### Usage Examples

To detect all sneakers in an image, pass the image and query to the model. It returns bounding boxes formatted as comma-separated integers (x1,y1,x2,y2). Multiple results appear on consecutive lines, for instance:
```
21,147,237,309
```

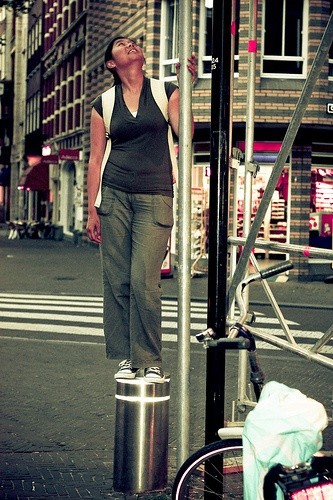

144,367,164,383
115,360,139,378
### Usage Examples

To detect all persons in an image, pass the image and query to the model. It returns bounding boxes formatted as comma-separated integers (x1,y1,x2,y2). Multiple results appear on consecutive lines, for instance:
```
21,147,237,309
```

85,37,199,384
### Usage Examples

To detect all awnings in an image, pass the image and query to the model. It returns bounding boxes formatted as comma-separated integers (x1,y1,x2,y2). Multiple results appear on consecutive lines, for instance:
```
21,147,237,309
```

17,161,50,191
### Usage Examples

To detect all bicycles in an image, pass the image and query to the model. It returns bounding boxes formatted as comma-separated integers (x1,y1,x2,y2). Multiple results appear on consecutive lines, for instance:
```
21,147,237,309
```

171,259,333,500
